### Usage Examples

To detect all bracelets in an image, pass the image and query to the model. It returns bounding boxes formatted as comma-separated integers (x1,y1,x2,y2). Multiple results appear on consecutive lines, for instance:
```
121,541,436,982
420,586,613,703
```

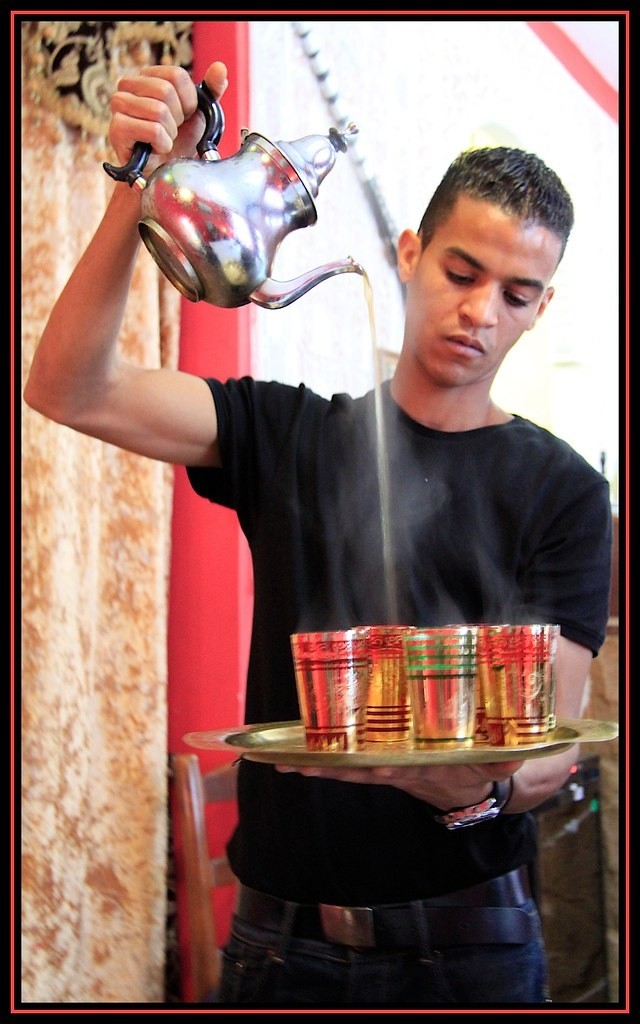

500,776,514,811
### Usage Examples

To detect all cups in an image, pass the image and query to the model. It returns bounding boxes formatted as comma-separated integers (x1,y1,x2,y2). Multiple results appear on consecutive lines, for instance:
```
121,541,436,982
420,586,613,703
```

289,621,561,754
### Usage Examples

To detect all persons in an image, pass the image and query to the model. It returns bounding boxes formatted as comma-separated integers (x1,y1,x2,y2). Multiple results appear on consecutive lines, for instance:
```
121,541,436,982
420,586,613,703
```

24,61,614,1003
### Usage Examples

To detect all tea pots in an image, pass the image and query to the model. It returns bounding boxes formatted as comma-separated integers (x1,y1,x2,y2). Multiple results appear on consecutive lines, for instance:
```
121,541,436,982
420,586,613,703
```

103,81,365,310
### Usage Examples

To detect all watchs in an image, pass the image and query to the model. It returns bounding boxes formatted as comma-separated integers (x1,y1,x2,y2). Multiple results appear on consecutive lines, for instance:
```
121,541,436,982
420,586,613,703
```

436,780,506,831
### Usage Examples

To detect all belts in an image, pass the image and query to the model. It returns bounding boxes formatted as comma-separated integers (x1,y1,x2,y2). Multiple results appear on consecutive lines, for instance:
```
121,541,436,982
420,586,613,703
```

233,863,533,951
